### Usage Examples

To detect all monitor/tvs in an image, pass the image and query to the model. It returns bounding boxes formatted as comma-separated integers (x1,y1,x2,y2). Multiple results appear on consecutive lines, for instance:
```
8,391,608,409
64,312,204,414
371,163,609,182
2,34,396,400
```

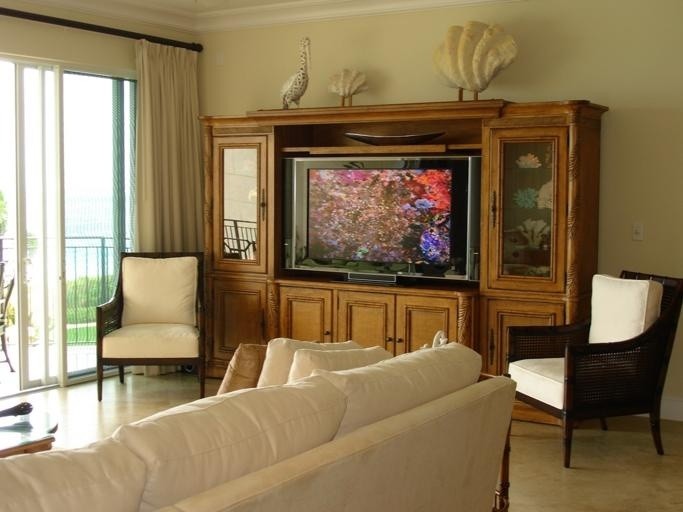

280,156,480,288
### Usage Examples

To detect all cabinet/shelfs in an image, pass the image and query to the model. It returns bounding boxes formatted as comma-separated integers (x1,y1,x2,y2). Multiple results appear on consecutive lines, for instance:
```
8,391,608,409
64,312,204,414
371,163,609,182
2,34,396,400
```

199,98,609,429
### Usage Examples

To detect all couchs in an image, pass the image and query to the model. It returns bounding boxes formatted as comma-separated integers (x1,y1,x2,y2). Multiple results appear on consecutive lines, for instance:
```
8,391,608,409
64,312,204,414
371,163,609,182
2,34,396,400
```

1,336,516,508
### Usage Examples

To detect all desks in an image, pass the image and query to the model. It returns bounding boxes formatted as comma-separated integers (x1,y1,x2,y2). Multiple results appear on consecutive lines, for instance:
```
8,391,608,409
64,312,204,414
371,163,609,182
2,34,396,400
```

2,408,53,464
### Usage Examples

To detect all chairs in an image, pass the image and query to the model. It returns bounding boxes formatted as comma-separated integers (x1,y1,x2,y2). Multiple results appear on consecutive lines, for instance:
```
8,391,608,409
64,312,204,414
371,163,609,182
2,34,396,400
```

94,249,209,408
497,269,683,468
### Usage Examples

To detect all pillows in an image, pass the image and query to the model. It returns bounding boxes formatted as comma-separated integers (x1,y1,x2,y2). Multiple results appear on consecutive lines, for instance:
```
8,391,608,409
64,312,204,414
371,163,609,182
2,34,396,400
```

211,338,392,407
